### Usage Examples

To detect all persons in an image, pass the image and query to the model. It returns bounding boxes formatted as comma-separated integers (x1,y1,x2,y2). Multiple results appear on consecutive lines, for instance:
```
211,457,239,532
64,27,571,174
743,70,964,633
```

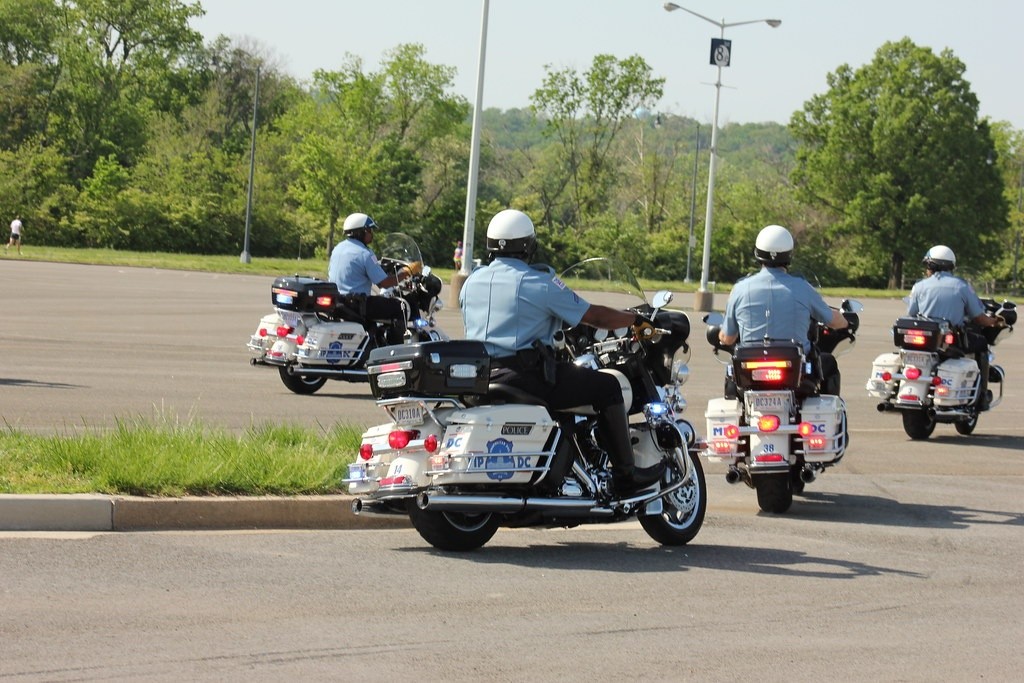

327,213,421,346
907,245,999,412
6,215,23,255
718,224,849,400
459,209,667,499
453,241,462,273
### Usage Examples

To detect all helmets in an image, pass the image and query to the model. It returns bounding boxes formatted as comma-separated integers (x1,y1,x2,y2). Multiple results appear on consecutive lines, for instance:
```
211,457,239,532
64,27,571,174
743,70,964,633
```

343,213,378,231
486,209,537,251
922,245,956,271
754,225,794,263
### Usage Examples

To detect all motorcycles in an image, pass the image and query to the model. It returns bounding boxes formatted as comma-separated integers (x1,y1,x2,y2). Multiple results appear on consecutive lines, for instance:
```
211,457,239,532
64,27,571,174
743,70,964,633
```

248,232,451,396
340,255,708,554
866,266,1019,439
702,264,865,515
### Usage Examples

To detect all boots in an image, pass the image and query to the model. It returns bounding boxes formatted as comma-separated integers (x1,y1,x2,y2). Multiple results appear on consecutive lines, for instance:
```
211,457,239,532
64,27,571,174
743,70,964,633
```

976,352,993,411
598,403,666,496
821,371,849,448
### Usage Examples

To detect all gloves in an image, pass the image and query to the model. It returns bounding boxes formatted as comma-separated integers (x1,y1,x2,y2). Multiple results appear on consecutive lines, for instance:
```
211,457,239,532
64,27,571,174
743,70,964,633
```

403,261,422,277
991,316,1005,329
633,312,656,340
843,312,860,333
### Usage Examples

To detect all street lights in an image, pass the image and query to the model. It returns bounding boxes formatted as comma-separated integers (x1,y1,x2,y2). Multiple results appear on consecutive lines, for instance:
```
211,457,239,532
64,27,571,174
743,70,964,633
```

664,1,782,311
